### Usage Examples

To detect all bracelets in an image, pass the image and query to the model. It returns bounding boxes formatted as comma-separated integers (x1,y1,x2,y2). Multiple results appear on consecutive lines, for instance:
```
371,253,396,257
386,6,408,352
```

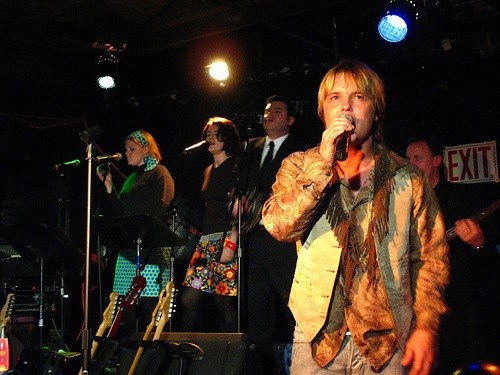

224,240,237,251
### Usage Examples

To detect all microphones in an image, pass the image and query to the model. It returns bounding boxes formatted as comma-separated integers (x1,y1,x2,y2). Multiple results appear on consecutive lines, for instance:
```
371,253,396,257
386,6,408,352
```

95,153,123,164
53,159,81,170
244,127,252,149
183,139,210,154
336,114,354,160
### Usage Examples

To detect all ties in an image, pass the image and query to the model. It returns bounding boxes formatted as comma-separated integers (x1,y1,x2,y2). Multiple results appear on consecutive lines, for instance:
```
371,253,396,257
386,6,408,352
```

259,140,274,175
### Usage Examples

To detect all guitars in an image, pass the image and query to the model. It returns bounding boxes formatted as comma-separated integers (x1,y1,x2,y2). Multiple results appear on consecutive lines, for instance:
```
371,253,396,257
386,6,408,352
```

0,275,178,375
444,199,500,241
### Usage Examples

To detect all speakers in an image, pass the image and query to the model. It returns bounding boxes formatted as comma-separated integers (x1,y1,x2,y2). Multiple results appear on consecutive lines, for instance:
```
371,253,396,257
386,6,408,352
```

13,348,82,375
115,331,266,375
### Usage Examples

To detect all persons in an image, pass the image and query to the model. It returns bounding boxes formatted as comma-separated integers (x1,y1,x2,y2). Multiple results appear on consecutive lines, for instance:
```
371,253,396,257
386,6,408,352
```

405,138,500,375
262,62,451,375
180,116,247,331
95,130,175,332
241,93,307,374
